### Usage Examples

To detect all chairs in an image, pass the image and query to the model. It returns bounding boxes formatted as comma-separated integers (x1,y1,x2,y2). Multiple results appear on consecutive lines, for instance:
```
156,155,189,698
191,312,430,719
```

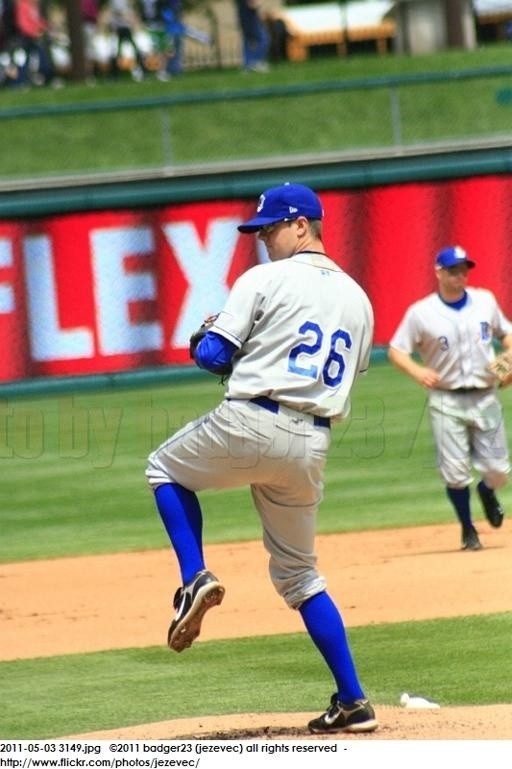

346,16,397,57
268,5,347,61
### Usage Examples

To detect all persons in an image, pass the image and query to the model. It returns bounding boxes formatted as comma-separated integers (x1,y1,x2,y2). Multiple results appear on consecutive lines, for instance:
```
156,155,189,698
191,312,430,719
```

387,244,512,551
145,182,381,734
0,0,186,88
238,0,293,78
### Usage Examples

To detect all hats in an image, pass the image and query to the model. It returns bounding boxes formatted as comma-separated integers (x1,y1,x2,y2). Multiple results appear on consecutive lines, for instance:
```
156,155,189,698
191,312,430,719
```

435,244,475,271
237,183,324,234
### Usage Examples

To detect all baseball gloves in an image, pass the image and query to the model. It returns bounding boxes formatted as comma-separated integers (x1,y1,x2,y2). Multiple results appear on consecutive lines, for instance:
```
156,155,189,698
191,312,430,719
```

486,352,512,388
189,313,230,385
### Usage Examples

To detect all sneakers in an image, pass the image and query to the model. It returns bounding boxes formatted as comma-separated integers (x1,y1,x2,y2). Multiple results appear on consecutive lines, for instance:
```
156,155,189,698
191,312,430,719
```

476,480,504,529
167,569,225,653
308,693,378,735
461,525,482,550
